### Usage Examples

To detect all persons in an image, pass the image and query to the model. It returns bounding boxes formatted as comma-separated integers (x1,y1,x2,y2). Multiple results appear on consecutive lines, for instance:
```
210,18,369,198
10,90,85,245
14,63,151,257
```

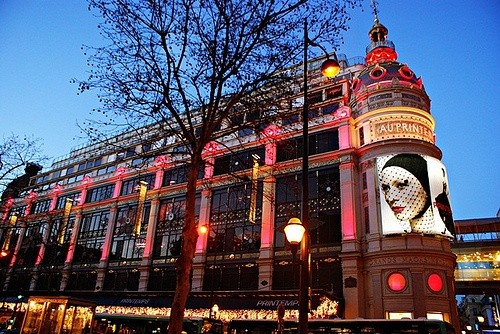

2,300,224,334
436,167,454,238
379,154,434,235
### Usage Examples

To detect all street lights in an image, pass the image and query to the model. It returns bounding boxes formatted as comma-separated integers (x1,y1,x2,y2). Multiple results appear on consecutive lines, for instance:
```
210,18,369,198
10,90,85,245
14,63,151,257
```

283,16,340,334
